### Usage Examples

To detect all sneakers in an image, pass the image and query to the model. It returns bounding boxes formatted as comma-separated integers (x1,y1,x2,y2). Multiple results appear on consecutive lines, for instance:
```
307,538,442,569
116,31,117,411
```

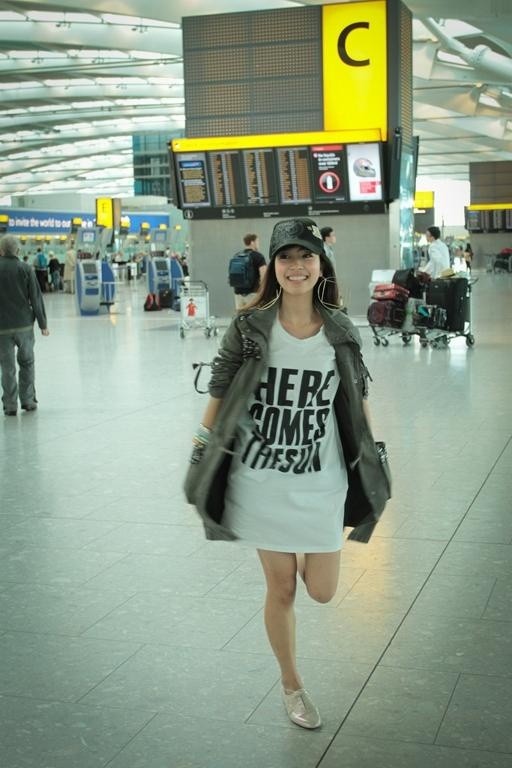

22,402,37,410
5,411,17,416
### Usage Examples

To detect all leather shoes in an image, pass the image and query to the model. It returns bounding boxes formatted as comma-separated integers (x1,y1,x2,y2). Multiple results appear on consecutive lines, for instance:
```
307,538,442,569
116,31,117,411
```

281,683,322,731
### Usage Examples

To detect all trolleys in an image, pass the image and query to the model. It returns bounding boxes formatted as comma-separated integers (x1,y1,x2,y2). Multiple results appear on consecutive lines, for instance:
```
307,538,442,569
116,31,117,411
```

174,278,220,338
369,323,414,346
414,273,480,349
484,251,505,274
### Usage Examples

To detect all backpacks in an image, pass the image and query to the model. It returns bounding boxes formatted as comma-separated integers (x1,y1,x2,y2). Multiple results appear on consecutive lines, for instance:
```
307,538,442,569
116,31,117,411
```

228,248,256,295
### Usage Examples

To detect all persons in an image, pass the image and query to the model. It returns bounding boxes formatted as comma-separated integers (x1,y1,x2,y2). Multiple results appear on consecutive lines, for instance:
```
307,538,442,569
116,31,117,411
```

0,232,49,417
319,225,337,277
184,218,396,730
21,241,191,295
421,243,473,274
233,233,268,318
418,225,453,281
185,298,199,316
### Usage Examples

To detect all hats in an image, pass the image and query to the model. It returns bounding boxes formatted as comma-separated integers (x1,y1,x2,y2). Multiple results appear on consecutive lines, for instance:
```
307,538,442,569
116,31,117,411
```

440,268,459,278
268,216,326,260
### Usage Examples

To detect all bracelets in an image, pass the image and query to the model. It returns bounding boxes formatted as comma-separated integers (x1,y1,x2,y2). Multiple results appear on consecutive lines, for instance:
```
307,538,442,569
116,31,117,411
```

198,422,214,434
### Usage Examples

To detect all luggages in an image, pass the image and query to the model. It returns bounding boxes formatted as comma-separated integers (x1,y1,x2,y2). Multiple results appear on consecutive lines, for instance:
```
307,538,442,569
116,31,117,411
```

366,266,469,334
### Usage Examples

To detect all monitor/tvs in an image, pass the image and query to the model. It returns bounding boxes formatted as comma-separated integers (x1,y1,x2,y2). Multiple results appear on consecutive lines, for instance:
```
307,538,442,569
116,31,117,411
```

155,229,166,241
155,261,167,271
82,264,97,273
81,229,96,243
